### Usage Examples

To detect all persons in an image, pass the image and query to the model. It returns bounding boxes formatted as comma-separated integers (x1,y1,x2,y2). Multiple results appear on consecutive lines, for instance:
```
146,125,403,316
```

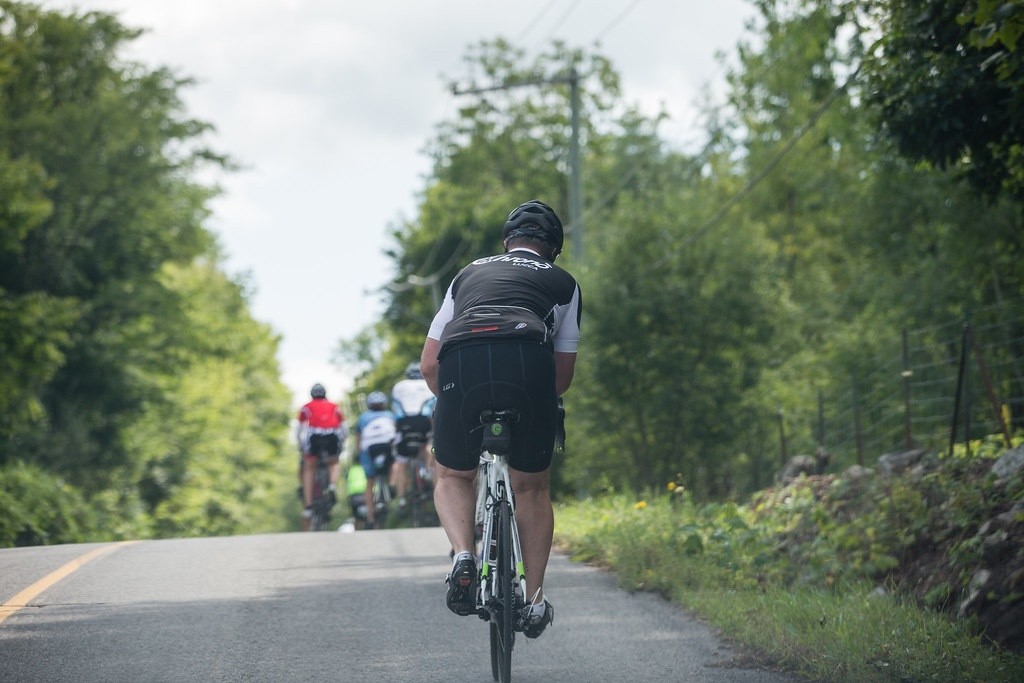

346,361,436,526
419,199,582,639
295,383,350,518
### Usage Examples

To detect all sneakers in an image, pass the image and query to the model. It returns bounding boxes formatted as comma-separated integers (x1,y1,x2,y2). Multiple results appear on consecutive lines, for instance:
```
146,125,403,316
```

444,551,476,618
522,597,554,639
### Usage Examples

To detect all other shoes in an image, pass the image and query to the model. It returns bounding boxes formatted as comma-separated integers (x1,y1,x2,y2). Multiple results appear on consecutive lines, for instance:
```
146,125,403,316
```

302,510,315,521
362,514,374,529
419,468,436,482
398,497,408,507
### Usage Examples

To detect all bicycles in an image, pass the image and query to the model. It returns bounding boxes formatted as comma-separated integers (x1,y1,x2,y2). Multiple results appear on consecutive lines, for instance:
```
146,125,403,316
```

367,430,431,530
453,408,528,683
303,451,337,532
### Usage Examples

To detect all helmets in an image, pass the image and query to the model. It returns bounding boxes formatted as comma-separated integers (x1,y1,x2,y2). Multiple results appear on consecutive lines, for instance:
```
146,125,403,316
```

366,391,390,411
312,383,327,400
406,361,425,381
501,199,563,252
